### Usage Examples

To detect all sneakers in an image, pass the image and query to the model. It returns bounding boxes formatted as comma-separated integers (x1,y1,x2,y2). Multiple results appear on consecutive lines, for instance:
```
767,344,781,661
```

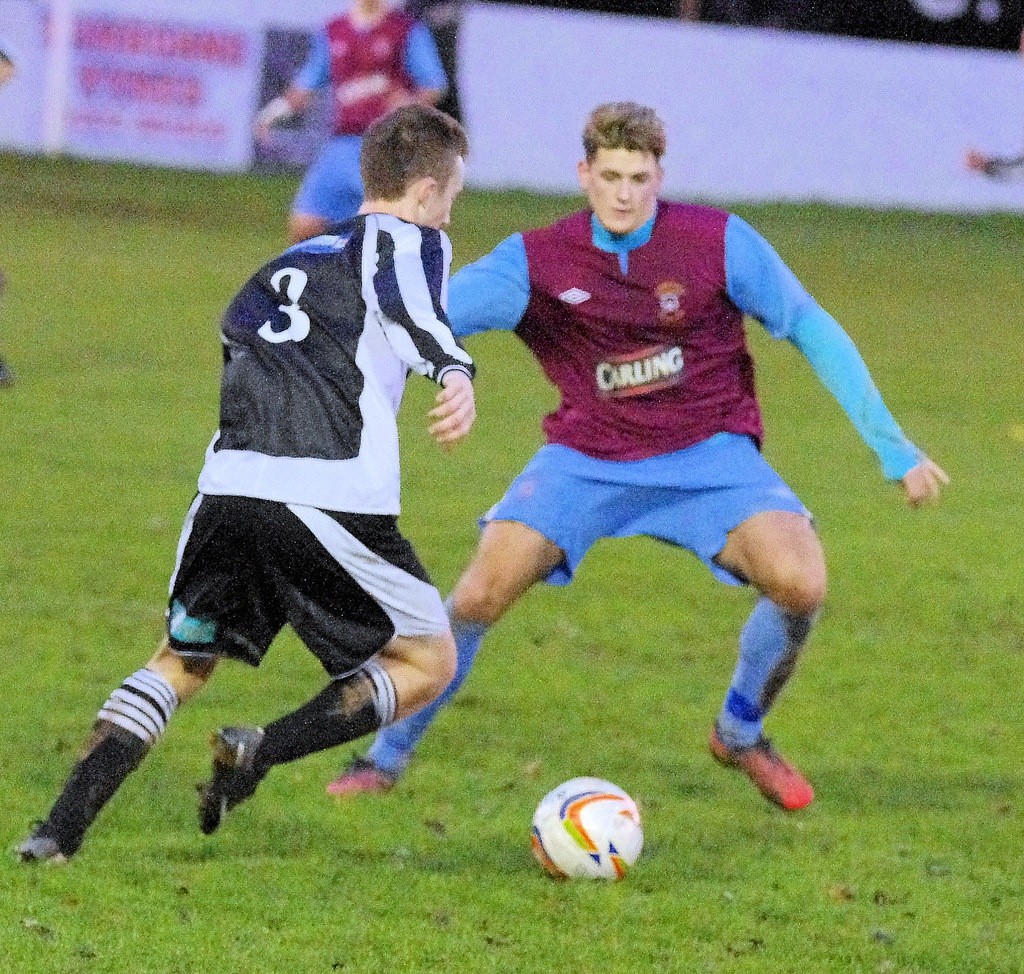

192,724,266,834
708,720,815,812
325,751,396,799
11,819,68,865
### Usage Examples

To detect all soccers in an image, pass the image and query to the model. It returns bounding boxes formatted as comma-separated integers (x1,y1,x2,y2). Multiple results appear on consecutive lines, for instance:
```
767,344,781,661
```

525,773,646,882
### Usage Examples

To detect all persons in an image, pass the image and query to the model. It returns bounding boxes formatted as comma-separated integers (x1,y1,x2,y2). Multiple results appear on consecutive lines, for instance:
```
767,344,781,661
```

0,49,14,386
323,103,952,810
964,149,1024,182
253,0,447,247
17,104,477,866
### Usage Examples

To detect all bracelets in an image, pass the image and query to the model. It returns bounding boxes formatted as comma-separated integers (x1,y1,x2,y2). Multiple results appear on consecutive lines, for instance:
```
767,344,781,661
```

259,97,294,125
